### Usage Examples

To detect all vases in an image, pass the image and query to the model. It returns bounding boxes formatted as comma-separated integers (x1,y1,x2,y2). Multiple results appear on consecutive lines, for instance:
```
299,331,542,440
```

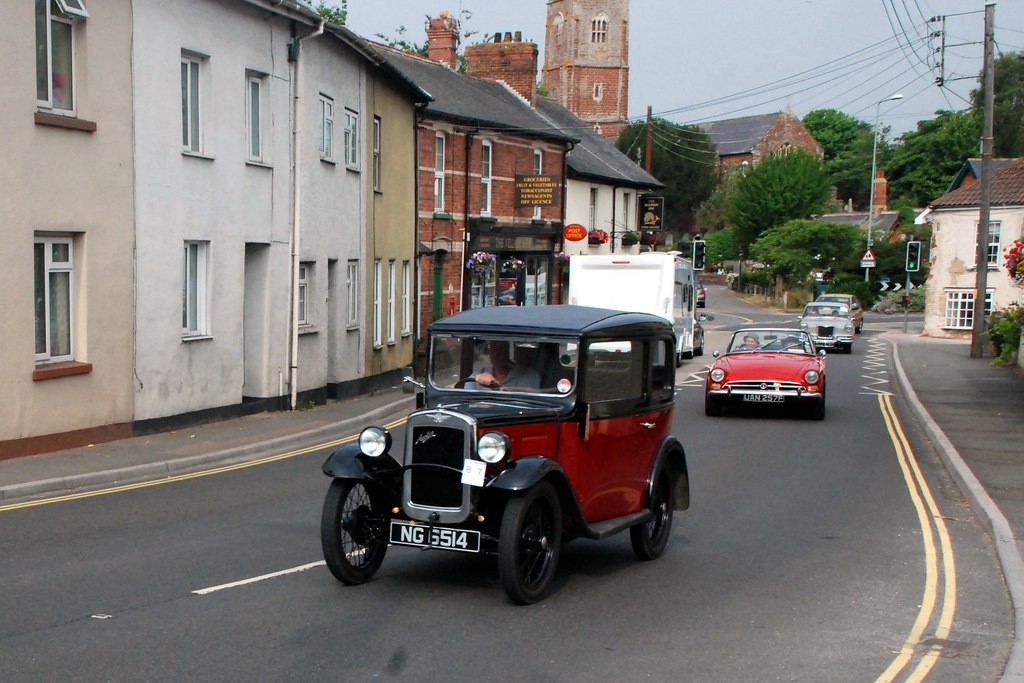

588,238,605,245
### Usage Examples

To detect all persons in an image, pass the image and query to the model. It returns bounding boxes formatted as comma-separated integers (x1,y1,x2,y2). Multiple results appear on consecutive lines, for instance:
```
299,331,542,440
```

778,334,806,354
732,333,761,351
833,310,839,317
446,341,542,393
811,307,820,314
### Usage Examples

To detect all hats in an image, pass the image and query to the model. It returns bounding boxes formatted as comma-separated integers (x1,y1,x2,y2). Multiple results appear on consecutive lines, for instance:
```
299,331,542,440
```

744,332,759,344
781,336,804,346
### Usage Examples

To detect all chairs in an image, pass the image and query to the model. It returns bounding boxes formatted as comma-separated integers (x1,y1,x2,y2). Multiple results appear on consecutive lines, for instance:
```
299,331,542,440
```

505,346,640,402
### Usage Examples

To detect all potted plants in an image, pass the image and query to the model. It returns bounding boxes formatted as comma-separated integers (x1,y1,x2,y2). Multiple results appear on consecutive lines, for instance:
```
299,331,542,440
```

984,302,1024,368
621,230,641,245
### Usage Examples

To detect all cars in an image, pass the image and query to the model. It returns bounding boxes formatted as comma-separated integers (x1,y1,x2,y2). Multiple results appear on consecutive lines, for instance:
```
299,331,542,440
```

798,302,856,354
814,294,865,334
703,326,826,421
697,282,707,308
321,304,690,606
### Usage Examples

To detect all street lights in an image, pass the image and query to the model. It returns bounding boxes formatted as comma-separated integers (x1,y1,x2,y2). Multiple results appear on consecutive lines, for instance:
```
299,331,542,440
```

864,93,904,281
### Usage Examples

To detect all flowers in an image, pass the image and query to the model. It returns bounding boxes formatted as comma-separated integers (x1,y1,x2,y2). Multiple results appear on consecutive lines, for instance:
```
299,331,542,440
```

588,229,609,245
500,256,525,275
464,252,497,275
1001,238,1024,281
554,251,570,264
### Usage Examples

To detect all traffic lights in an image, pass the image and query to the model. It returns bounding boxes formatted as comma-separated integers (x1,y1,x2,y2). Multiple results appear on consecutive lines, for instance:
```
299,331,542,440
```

693,240,705,271
905,241,921,273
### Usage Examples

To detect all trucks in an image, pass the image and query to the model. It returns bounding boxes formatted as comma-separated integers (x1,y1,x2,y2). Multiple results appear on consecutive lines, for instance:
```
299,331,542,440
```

568,251,704,369
